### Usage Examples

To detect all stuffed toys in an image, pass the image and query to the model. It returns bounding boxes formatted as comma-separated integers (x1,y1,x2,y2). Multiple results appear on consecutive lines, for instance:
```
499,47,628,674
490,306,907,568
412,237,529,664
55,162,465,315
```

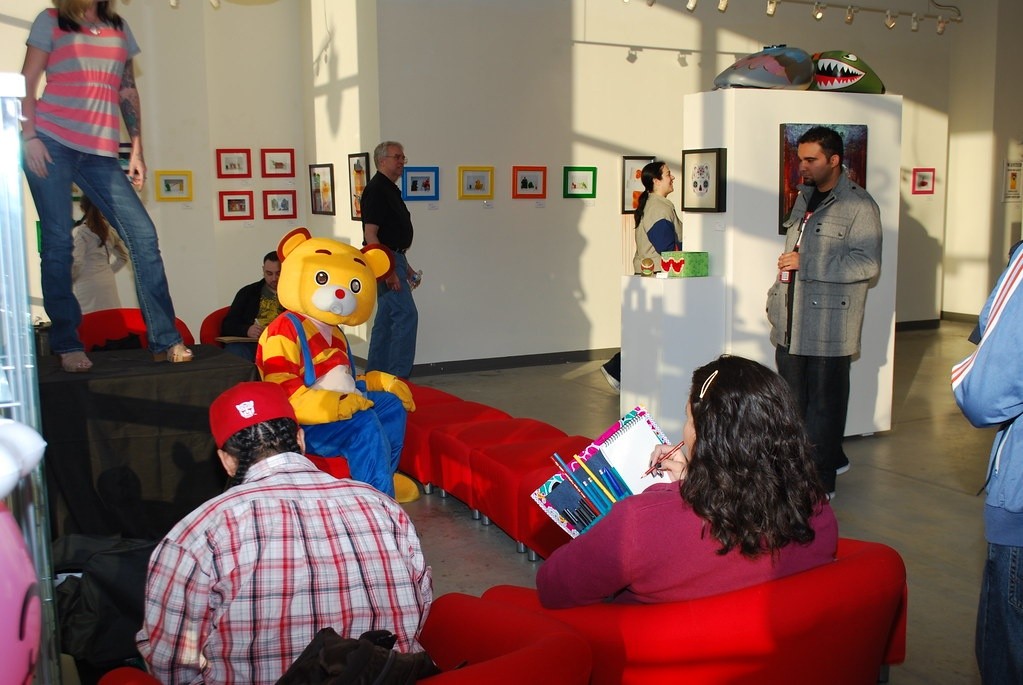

254,224,420,501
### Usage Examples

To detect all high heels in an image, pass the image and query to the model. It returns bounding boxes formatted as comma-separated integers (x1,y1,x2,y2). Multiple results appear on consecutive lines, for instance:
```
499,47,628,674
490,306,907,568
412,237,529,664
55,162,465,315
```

152,341,192,363
62,352,90,372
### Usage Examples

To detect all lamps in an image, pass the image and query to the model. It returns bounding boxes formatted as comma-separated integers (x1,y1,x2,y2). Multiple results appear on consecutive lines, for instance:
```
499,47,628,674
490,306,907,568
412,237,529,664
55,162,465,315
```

624,0,963,36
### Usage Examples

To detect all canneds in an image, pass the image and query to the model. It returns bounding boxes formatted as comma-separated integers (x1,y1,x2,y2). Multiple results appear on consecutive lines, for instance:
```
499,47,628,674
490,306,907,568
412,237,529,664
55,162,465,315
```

779,269,792,284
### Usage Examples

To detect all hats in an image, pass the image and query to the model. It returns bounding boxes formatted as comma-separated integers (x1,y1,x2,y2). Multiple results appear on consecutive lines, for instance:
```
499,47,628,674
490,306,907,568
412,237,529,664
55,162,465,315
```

209,381,300,450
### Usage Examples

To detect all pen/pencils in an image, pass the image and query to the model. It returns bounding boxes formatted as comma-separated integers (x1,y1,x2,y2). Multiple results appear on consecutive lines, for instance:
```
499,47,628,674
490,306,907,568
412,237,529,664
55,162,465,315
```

550,453,624,532
641,441,684,479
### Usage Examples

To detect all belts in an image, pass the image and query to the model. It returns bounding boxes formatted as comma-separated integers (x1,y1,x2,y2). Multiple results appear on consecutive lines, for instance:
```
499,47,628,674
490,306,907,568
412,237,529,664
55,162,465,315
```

394,249,406,254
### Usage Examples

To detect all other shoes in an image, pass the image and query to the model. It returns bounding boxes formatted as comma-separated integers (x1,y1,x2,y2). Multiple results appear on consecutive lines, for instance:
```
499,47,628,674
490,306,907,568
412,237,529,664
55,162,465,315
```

835,455,850,475
601,366,620,393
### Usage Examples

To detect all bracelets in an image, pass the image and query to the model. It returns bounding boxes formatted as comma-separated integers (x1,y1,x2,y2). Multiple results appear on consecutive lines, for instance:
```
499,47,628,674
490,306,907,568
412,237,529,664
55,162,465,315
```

23,134,38,141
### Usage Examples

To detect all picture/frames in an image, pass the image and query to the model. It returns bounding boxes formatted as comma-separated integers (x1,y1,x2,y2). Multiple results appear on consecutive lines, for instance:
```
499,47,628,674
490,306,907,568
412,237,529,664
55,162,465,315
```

308,164,335,215
911,168,935,194
458,166,494,201
681,148,728,213
216,149,251,179
622,155,656,215
263,190,297,219
218,191,255,220
154,170,193,202
562,166,597,198
512,165,547,199
261,148,295,178
69,142,149,202
348,152,371,221
400,166,440,201
1000,159,1023,204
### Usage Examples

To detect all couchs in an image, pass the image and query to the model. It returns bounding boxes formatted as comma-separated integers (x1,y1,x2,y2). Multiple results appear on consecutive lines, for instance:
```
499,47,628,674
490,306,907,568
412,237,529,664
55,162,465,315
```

70,306,910,684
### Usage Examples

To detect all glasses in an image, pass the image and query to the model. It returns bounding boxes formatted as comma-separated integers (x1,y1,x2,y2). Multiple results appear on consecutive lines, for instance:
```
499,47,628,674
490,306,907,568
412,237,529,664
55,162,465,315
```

384,155,408,164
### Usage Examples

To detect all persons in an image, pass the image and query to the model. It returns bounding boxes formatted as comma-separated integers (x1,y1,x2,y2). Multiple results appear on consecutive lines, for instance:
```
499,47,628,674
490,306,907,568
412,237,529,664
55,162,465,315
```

599,160,683,392
765,124,884,499
950,232,1023,685
360,139,419,379
537,352,841,609
20,0,195,372
68,190,130,314
219,249,288,381
135,380,437,685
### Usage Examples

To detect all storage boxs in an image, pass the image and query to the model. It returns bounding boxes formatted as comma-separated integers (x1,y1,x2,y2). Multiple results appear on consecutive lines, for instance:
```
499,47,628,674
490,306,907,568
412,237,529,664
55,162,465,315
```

661,251,709,277
